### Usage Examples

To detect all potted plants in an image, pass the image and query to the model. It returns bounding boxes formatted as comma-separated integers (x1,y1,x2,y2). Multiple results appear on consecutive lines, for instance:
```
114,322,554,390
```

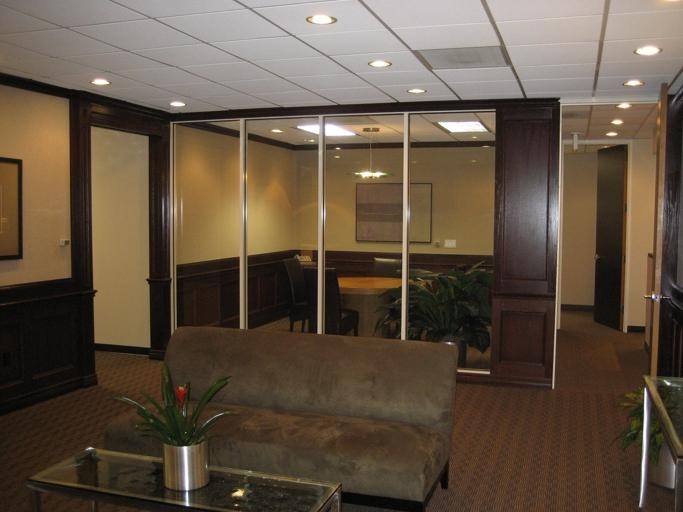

373,260,493,369
616,371,683,489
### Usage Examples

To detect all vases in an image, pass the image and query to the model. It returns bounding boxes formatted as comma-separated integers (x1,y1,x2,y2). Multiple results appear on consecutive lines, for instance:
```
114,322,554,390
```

164,442,210,491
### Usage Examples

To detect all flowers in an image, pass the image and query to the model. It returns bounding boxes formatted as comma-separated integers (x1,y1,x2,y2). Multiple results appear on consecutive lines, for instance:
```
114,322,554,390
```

111,362,240,446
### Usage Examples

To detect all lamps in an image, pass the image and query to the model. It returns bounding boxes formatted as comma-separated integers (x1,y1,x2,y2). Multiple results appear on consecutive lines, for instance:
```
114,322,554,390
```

355,128,388,179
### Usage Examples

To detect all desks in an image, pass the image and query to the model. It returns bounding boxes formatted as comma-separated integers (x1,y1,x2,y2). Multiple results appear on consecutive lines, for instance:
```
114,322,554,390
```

638,374,683,512
337,277,411,337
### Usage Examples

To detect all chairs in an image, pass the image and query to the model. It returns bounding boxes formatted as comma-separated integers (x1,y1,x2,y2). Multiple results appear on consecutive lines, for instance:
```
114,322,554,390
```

282,258,312,331
304,268,359,336
372,257,402,278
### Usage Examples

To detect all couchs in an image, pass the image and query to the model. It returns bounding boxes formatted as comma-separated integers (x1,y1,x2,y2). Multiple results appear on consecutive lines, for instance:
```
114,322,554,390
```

103,325,460,512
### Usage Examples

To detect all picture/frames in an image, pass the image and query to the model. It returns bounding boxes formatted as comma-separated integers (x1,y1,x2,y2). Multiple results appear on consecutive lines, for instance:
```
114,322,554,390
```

355,182,432,244
0,157,23,260
